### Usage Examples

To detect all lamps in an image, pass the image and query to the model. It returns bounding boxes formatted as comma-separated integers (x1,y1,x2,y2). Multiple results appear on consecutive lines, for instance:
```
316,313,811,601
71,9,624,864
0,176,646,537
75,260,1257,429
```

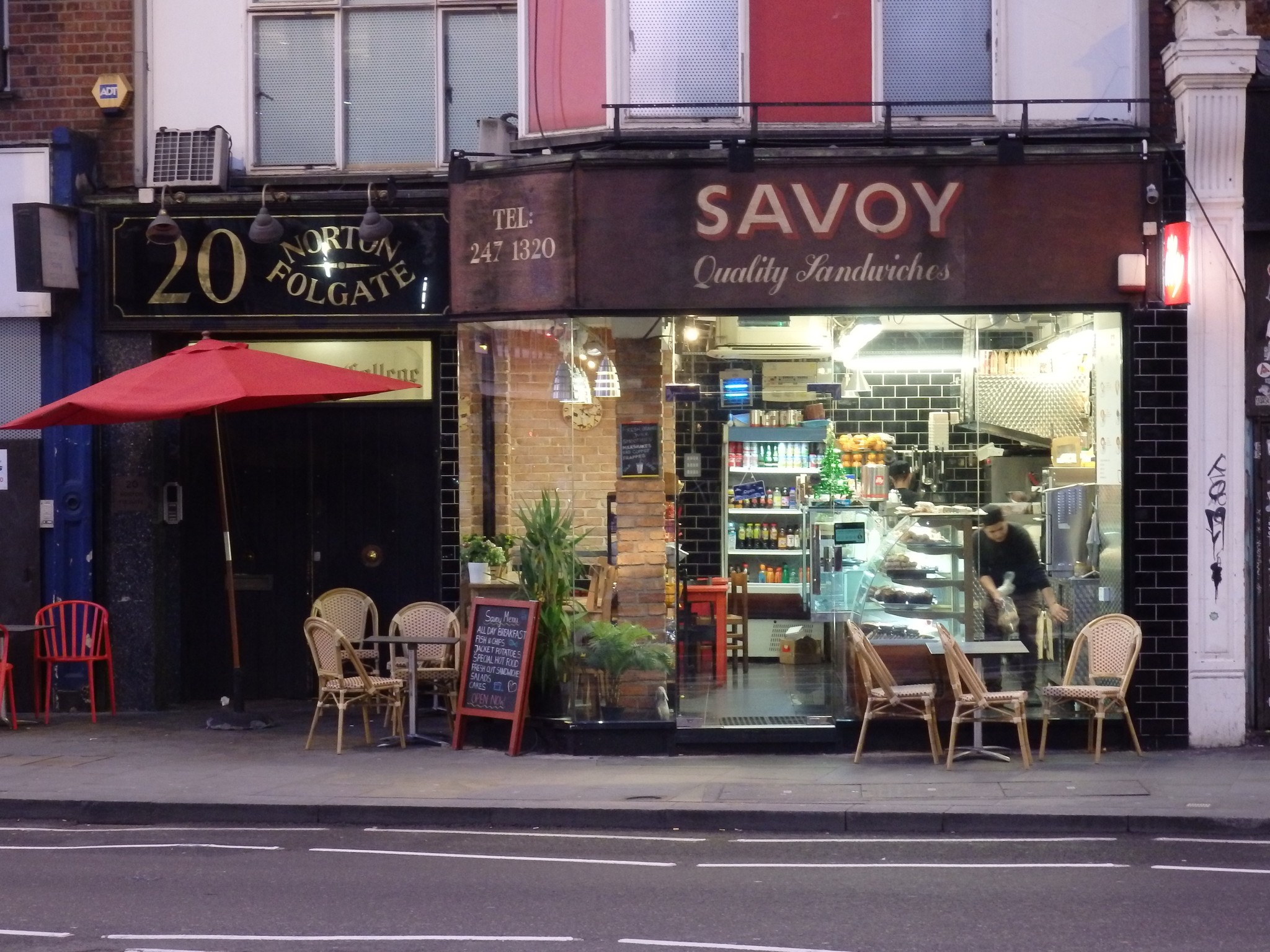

725,137,826,173
567,358,593,404
552,326,580,401
949,131,1026,168
358,181,394,243
248,182,289,244
147,184,187,245
559,345,586,404
447,148,534,184
595,318,621,398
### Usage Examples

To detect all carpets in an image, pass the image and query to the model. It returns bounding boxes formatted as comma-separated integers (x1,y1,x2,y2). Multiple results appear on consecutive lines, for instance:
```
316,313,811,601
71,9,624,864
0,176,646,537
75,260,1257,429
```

719,716,808,725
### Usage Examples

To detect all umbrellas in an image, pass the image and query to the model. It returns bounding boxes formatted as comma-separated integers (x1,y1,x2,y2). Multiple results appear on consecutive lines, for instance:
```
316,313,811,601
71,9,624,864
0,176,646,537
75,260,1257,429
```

0,331,426,729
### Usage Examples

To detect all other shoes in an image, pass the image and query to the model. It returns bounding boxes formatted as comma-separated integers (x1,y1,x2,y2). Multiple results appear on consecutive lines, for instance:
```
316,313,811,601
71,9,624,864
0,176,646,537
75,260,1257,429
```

984,703,1003,710
1024,691,1042,707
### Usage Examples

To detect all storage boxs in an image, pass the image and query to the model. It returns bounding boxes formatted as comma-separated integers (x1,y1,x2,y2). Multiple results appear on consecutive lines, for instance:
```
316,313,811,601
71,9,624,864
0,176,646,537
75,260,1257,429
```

779,635,822,665
784,625,806,641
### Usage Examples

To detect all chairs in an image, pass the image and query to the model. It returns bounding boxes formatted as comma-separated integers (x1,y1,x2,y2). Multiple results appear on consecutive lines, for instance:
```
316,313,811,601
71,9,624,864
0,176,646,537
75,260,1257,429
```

383,600,460,737
34,599,119,724
584,562,603,609
1038,612,1143,767
562,565,616,706
308,588,381,717
678,610,698,682
935,622,1033,772
845,618,946,767
302,616,407,755
699,570,748,675
0,623,18,730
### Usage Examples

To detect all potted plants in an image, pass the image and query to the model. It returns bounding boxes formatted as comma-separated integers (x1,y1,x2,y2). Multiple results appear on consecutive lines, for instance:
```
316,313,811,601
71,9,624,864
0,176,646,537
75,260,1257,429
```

482,485,598,718
556,617,678,721
491,532,516,577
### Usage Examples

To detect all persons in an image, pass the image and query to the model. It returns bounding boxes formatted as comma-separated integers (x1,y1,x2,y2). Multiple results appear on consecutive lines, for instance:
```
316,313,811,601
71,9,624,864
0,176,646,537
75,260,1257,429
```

969,505,1069,705
871,462,922,513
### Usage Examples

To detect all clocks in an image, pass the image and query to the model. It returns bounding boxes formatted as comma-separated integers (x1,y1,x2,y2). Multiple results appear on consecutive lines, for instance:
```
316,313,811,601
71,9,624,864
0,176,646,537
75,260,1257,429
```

561,397,602,431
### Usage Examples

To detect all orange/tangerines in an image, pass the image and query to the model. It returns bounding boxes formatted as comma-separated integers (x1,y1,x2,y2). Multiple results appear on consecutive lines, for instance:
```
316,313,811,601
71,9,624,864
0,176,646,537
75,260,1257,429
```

838,433,886,466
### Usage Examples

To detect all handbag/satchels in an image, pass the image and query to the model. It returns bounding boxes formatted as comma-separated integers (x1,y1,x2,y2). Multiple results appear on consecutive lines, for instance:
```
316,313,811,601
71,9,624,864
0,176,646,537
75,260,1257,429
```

993,569,1019,634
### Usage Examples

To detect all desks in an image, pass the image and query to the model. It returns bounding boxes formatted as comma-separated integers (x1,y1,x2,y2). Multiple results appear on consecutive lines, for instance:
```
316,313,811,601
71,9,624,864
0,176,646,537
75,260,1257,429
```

686,586,731,687
363,635,461,750
924,641,1030,762
0,625,55,729
468,571,538,607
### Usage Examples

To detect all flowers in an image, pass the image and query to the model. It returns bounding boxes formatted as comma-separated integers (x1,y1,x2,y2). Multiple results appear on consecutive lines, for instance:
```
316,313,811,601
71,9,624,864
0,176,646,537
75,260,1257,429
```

460,533,507,566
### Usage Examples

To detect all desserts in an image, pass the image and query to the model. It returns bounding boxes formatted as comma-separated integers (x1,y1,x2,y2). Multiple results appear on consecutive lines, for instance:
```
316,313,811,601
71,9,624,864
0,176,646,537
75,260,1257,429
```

867,584,934,604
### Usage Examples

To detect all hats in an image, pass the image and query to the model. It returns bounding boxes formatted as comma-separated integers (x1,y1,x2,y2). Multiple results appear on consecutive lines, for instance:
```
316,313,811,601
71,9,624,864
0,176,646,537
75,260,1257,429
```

980,505,1004,526
889,460,911,476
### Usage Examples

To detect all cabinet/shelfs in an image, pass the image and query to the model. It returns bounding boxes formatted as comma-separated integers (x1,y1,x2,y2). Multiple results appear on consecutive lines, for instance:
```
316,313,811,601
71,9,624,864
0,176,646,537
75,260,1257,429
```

847,513,973,718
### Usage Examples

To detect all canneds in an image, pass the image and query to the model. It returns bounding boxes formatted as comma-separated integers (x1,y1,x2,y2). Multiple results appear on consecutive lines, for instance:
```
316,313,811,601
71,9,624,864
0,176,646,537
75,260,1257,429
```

749,409,802,427
777,442,824,468
728,442,778,467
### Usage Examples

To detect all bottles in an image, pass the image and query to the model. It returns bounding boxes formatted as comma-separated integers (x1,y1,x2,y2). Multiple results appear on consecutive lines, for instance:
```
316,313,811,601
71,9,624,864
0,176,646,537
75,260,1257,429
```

728,522,811,550
727,483,798,510
759,562,810,584
728,563,750,583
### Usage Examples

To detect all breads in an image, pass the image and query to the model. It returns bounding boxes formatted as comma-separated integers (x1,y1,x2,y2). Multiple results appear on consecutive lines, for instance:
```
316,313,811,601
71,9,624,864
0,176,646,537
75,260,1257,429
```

898,526,947,545
884,553,917,570
990,349,1039,375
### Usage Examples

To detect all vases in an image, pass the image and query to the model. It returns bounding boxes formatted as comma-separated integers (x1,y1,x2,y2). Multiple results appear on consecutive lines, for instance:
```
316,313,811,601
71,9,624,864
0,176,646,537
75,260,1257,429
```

468,562,491,583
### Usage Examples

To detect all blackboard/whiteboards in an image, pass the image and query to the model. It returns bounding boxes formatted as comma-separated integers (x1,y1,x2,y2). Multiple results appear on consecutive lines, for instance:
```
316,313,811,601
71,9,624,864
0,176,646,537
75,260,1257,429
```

733,480,766,501
457,597,542,721
621,424,659,477
